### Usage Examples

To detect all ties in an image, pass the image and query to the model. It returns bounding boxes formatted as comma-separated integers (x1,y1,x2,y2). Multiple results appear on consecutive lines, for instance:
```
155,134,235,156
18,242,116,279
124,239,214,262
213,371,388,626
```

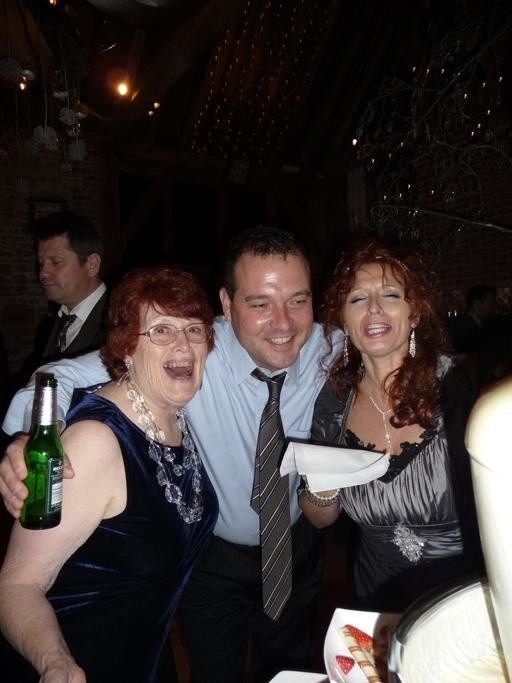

248,368,293,623
49,311,78,354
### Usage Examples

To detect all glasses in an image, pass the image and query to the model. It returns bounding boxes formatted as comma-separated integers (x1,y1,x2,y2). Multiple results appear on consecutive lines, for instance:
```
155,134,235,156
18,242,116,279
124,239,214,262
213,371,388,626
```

131,321,216,346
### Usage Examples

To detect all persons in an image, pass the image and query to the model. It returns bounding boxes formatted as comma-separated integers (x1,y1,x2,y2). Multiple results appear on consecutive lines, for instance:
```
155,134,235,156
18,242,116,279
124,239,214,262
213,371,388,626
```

442,283,503,351
293,237,489,617
1,263,223,682
31,210,115,364
1,224,353,682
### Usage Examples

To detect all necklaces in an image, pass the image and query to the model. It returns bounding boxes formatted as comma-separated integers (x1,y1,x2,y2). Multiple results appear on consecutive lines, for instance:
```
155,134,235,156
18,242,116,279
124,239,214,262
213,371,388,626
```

356,372,417,451
127,370,193,476
126,379,204,525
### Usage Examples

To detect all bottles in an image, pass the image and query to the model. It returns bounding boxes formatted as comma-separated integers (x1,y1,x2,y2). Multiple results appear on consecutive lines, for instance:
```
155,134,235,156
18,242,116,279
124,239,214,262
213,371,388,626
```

18,372,66,531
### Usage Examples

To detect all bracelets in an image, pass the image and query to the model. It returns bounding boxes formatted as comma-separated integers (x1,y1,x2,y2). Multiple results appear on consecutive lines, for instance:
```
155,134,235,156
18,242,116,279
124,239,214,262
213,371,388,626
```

301,481,342,507
